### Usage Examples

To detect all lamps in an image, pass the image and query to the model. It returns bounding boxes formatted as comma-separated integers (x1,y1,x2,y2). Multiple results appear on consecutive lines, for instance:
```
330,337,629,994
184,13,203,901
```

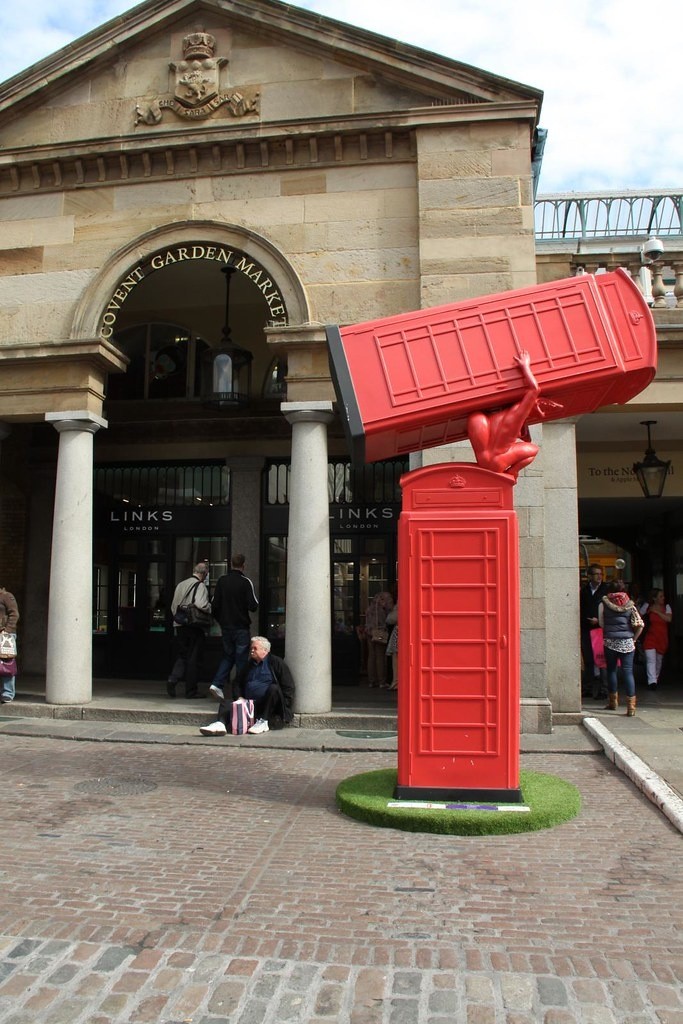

633,420,672,499
202,267,250,409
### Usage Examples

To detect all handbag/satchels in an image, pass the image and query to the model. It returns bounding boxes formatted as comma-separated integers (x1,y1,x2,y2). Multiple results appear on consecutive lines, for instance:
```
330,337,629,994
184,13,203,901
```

233,696,254,734
371,629,389,644
0,631,18,676
174,582,213,628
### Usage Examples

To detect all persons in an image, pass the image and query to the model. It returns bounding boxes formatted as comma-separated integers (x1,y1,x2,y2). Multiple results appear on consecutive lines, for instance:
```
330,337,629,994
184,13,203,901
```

0,584,19,703
200,636,295,736
335,618,353,633
167,563,211,699
639,588,673,690
208,553,259,702
364,591,398,690
580,563,647,716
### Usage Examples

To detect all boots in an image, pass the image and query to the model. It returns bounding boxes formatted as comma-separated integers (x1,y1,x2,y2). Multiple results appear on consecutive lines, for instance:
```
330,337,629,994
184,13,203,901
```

605,691,619,710
627,695,636,717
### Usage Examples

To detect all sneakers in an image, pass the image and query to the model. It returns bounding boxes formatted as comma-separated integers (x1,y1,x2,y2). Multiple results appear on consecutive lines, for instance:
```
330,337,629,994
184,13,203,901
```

200,721,228,736
247,719,270,734
210,685,224,700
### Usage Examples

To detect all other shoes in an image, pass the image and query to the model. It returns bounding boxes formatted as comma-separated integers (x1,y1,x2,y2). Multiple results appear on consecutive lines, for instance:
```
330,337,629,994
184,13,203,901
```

1,696,12,703
595,692,606,699
582,690,593,697
187,692,206,698
166,681,177,698
649,683,658,691
369,682,391,689
